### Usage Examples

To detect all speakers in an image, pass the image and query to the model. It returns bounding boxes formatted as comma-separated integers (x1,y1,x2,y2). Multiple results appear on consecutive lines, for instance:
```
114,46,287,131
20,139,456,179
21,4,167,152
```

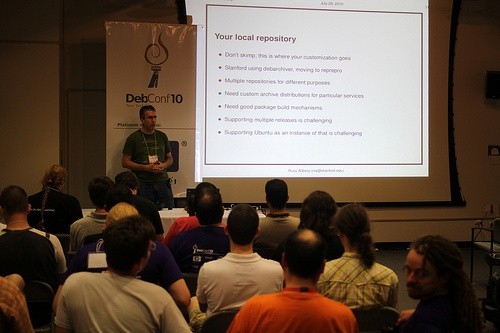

485,71,500,99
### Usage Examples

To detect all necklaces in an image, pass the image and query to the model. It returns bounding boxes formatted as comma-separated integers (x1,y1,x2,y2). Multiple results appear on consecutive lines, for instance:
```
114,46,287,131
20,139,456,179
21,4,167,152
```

7,220,27,227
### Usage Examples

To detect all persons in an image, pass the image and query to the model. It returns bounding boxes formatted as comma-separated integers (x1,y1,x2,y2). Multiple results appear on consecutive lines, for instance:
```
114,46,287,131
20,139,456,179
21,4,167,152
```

190,206,284,324
25,164,83,240
104,184,136,216
51,215,194,333
244,179,303,262
272,191,345,271
391,234,487,333
167,188,243,295
49,201,192,316
114,169,164,239
162,181,228,252
227,227,360,333
0,184,67,331
68,174,115,255
0,273,35,333
120,105,182,212
313,203,398,316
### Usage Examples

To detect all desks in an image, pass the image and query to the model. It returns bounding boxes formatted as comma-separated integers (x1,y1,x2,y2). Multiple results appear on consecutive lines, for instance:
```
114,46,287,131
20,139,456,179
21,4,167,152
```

81,208,266,238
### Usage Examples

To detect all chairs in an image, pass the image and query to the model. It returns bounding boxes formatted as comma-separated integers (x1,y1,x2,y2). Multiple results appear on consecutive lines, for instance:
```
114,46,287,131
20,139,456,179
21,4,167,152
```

0,234,402,333
470,218,500,282
